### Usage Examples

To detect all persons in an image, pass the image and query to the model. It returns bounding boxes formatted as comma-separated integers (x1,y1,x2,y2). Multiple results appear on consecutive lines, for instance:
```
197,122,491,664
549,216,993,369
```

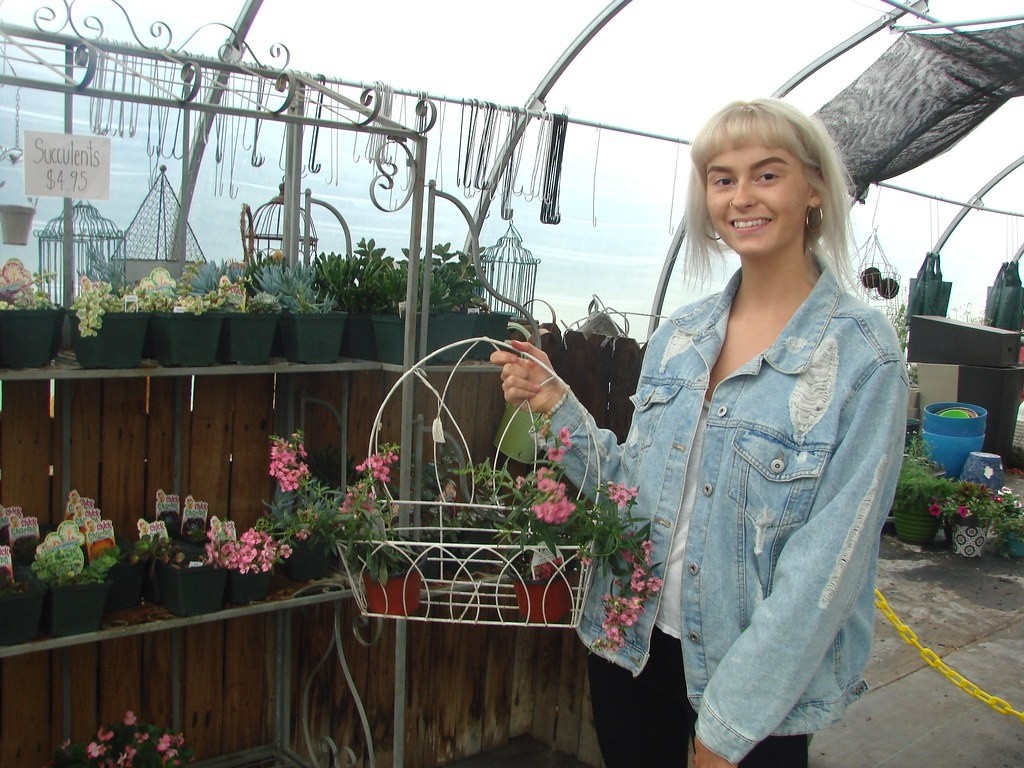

490,97,909,767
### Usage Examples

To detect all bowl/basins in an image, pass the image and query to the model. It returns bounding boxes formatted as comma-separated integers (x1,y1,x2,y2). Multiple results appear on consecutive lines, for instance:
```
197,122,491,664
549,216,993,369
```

934,407,978,419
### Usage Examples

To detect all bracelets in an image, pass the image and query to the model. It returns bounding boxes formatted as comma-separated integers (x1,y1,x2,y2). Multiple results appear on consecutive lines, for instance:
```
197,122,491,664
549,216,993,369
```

543,385,570,419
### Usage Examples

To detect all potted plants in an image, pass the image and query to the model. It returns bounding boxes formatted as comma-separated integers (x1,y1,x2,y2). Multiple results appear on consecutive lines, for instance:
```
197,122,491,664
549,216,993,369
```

152,532,227,616
0,243,520,370
0,533,150,648
891,460,939,546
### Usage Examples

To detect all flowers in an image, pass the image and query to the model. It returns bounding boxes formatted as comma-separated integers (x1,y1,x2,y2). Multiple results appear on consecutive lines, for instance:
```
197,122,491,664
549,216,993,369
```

55,710,196,768
925,475,1024,559
450,400,667,650
205,430,425,585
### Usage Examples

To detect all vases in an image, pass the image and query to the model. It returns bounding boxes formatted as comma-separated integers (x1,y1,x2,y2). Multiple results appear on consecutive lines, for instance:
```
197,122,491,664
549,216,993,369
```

362,534,425,615
502,538,570,623
953,523,989,558
905,315,1024,489
0,205,35,246
276,529,330,581
223,569,269,603
1006,530,1024,557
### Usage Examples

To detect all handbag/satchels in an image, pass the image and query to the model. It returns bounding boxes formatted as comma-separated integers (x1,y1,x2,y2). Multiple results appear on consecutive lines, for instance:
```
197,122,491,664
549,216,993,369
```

906,253,952,325
984,262,1024,331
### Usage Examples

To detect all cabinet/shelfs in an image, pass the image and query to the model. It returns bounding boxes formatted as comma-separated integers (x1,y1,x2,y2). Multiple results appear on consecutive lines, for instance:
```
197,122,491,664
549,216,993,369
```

0,363,526,768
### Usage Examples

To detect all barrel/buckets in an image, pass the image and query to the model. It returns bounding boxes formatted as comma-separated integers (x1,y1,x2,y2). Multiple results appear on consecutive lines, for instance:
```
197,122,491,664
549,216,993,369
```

905,400,1005,490
